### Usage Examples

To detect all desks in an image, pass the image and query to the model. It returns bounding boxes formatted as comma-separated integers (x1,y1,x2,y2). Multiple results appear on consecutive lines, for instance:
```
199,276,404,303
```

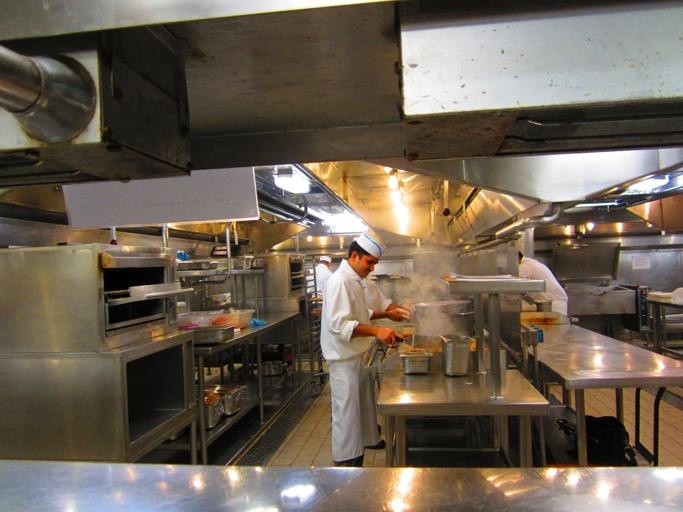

521,323,683,466
152,310,301,465
378,337,551,468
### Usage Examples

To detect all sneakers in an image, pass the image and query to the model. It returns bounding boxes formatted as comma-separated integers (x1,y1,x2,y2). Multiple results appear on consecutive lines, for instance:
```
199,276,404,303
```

364,439,385,448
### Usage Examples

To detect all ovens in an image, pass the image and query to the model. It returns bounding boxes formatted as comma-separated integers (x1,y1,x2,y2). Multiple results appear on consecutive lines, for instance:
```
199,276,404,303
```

233,252,308,298
1,243,178,356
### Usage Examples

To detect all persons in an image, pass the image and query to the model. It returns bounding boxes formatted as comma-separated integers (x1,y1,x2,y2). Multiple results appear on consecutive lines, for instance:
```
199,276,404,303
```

320,232,411,466
518,250,569,317
306,255,333,304
363,276,411,433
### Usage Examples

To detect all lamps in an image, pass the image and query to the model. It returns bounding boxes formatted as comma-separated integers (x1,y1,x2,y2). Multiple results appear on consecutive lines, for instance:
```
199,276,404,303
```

273,174,309,194
388,169,398,190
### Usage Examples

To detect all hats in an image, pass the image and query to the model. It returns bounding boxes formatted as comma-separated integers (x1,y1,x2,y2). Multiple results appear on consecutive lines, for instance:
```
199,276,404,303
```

320,255,331,264
357,233,385,259
